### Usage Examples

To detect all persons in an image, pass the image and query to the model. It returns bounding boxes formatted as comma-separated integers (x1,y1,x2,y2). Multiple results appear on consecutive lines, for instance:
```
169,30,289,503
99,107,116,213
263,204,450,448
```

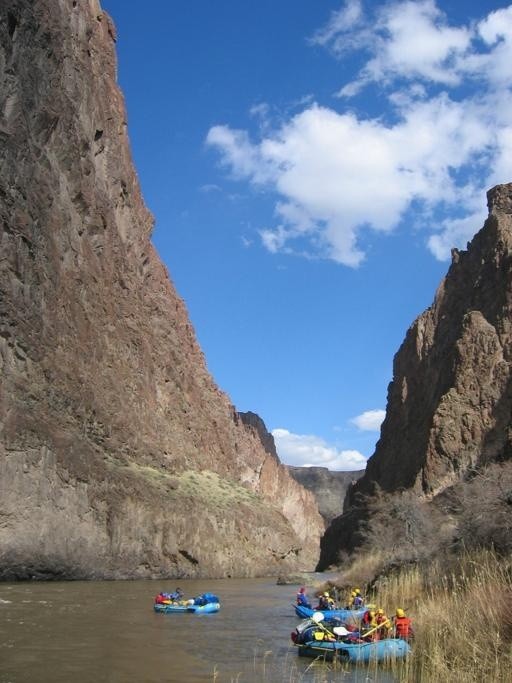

155,587,185,606
362,608,415,644
296,587,363,609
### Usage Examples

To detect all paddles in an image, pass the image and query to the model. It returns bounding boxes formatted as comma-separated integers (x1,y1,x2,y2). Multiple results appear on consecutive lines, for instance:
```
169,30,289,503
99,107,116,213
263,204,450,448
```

362,608,409,638
291,604,335,636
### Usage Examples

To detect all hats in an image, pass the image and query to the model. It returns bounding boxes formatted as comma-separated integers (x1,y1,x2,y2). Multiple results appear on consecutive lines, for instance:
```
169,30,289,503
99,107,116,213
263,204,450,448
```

352,588,360,598
396,609,405,618
324,592,329,597
370,609,383,614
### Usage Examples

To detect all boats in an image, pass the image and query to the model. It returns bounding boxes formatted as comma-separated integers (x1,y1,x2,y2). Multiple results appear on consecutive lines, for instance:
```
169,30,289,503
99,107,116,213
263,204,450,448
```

153,598,221,614
296,633,412,665
295,604,374,623
